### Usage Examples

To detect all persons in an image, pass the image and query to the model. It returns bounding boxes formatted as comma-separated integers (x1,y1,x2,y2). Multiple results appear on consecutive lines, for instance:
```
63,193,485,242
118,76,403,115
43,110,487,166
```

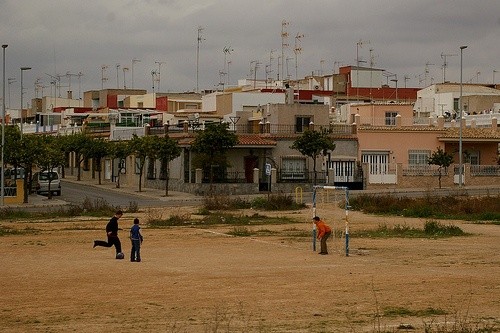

93,210,123,259
313,216,331,255
130,218,143,262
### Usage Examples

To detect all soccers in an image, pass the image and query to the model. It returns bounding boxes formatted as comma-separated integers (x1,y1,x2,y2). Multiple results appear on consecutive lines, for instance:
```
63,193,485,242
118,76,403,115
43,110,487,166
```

116,253,124,259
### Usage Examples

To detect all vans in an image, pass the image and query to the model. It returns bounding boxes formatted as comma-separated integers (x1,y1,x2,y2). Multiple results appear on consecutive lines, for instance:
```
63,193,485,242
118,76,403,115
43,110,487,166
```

31,170,61,196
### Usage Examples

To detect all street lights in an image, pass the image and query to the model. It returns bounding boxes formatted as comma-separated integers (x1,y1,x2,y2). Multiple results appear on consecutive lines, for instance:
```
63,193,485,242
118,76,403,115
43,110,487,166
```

1,45,8,207
459,46,467,187
20,67,31,140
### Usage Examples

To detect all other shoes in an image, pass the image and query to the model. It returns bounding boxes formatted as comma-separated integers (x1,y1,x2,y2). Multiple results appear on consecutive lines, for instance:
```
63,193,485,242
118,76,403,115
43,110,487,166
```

318,251,322,254
136,258,141,262
131,259,136,262
322,252,328,255
93,240,96,248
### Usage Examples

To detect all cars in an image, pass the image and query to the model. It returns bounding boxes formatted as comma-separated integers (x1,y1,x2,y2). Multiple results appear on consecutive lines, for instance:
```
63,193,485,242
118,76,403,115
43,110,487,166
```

5,168,26,179
